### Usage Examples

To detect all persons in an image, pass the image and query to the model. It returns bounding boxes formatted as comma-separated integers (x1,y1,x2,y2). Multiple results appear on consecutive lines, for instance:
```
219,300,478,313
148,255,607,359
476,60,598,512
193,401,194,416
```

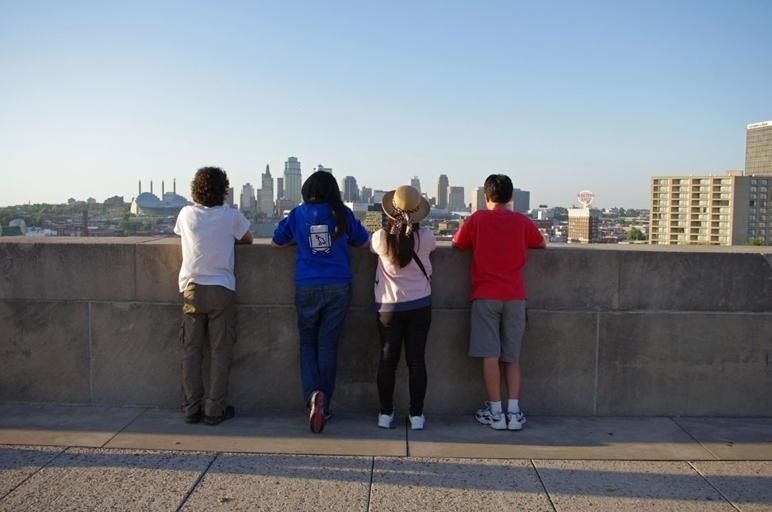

271,171,370,434
369,185,440,431
171,166,254,426
449,172,549,431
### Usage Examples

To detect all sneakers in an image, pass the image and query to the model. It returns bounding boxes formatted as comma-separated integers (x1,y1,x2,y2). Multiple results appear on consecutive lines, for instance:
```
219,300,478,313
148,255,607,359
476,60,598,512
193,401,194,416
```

409,411,425,429
204,405,235,425
378,400,395,428
304,389,333,433
184,404,202,423
506,407,528,430
475,408,507,430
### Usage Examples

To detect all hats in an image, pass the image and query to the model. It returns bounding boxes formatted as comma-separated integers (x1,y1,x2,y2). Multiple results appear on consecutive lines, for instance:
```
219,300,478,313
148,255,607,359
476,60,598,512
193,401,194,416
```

381,186,430,224
